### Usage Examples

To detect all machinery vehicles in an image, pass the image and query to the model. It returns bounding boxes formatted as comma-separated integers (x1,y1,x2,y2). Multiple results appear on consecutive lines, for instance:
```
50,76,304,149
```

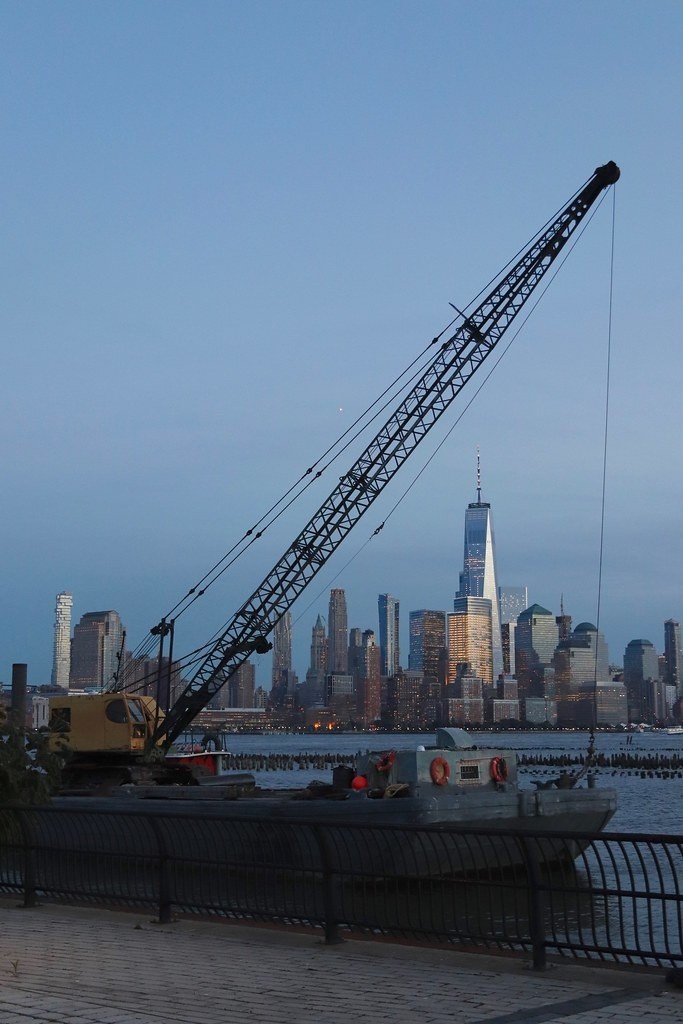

31,157,622,780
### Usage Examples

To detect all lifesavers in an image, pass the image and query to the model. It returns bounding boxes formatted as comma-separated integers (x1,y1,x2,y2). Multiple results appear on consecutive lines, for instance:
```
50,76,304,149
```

429,758,450,784
491,756,509,781
376,752,395,771
201,733,221,753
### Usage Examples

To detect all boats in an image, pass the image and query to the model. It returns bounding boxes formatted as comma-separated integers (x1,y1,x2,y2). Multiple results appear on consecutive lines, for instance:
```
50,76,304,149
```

666,725,683,734
0,157,620,882
